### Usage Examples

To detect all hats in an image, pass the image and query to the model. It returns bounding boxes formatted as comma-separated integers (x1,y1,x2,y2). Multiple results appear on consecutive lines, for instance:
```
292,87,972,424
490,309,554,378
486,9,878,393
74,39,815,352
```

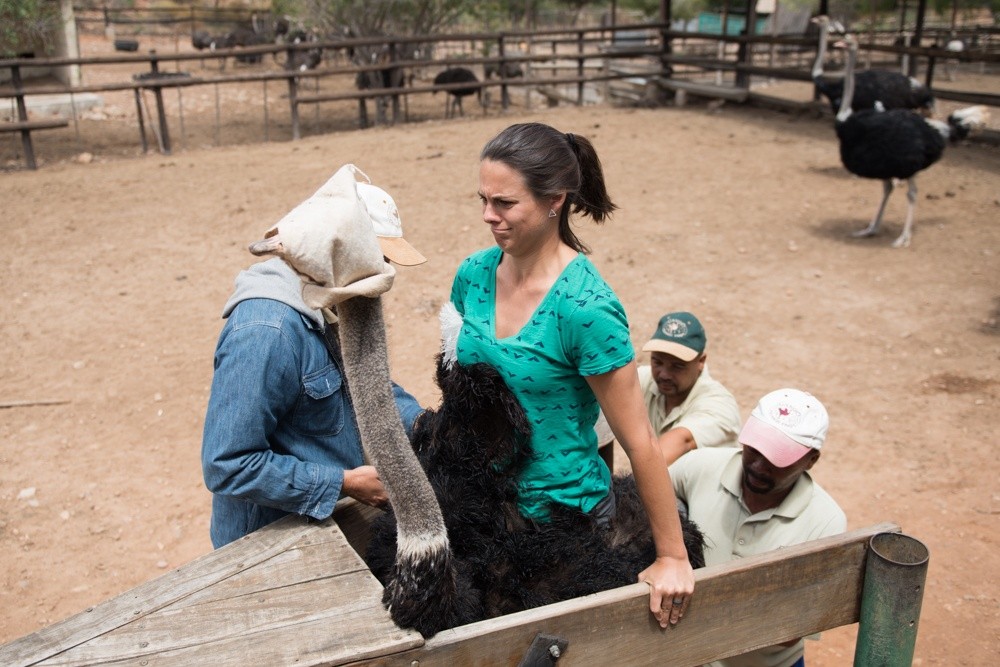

356,182,428,265
642,312,706,361
739,388,828,468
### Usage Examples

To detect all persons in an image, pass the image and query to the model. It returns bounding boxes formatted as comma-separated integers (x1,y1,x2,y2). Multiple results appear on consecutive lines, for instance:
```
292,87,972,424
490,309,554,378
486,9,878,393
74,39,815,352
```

636,311,741,468
667,388,847,667
450,122,695,629
201,163,427,546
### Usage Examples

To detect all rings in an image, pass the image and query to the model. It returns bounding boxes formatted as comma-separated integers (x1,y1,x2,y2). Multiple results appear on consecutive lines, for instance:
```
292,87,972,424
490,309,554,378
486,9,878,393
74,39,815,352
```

674,598,683,605
